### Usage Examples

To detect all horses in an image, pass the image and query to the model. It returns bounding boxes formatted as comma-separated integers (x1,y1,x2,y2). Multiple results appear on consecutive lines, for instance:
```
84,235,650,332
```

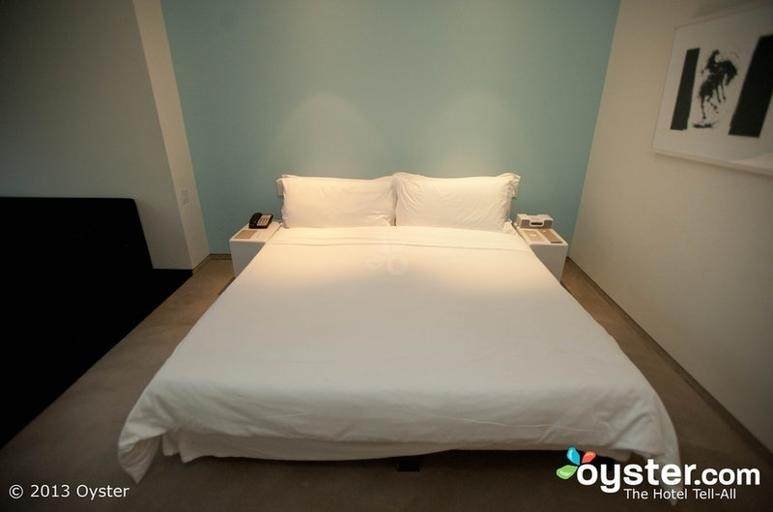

697,59,738,120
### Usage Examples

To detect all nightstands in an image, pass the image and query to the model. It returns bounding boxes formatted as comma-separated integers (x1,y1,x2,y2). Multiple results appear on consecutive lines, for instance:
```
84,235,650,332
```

514,213,568,283
228,221,280,277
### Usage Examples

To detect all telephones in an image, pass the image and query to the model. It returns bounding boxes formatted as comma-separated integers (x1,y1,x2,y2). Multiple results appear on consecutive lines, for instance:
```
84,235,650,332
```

249,213,273,228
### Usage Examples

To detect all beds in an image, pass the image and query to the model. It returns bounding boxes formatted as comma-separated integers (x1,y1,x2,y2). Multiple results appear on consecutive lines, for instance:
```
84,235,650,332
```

117,222,682,506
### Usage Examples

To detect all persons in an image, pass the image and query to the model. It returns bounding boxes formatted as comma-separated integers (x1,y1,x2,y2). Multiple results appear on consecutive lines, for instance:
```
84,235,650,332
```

702,48,722,78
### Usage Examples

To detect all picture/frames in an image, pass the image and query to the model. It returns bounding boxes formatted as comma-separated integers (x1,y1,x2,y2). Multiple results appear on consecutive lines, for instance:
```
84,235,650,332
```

649,0,773,178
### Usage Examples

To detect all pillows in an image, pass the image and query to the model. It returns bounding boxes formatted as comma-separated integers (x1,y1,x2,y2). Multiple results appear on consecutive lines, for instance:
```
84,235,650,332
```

394,173,520,230
275,174,396,229
